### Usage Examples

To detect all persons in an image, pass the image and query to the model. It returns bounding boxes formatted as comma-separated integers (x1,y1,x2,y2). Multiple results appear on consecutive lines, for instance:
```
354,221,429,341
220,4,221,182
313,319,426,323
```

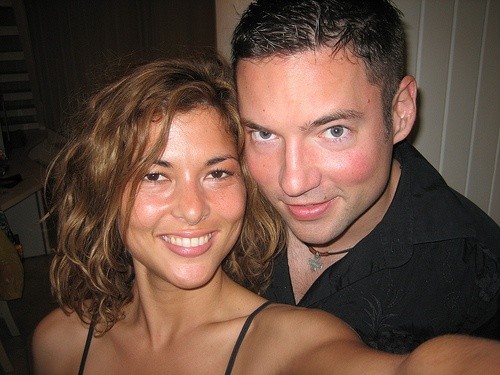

221,0,500,355
32,56,500,375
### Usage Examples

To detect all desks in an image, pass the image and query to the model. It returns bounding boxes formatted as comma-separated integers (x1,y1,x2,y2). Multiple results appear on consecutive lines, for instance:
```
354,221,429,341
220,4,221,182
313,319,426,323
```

1,142,47,259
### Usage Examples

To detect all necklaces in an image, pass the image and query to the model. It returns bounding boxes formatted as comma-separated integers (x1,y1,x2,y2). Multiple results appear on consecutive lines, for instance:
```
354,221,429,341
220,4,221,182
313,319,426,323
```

308,245,352,273
